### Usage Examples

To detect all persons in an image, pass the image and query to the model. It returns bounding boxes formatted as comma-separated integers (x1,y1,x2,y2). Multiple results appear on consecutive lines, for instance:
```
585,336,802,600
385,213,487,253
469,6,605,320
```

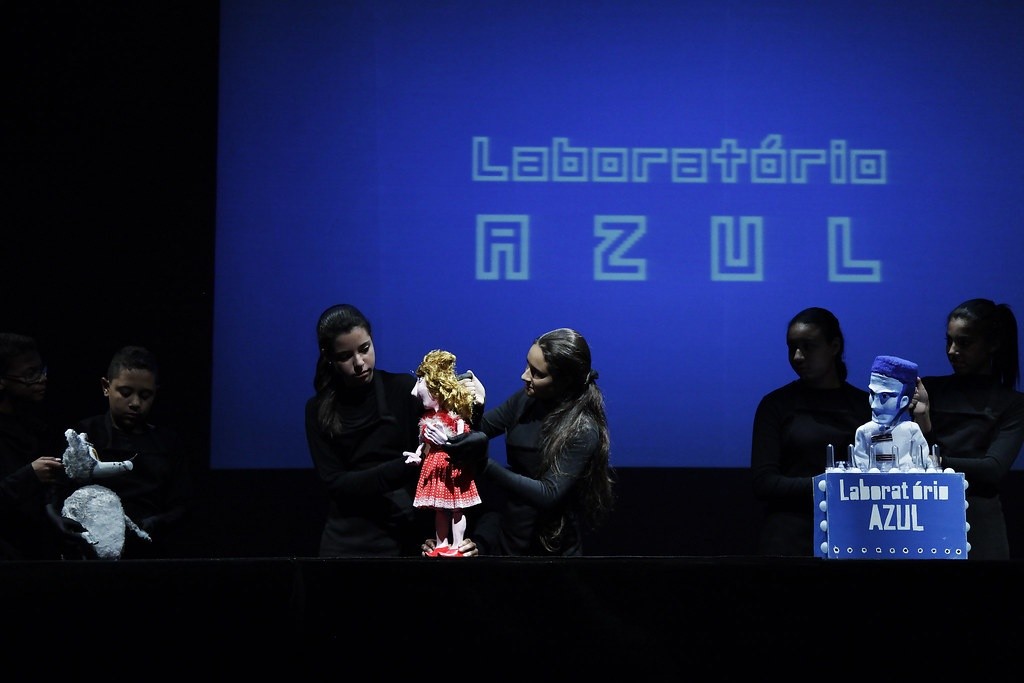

403,349,475,557
0,333,193,559
854,355,929,471
304,304,609,557
751,300,1024,552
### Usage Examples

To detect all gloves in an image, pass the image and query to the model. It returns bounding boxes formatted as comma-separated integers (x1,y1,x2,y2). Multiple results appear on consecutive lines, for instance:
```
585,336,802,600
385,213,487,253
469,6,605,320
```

444,432,489,458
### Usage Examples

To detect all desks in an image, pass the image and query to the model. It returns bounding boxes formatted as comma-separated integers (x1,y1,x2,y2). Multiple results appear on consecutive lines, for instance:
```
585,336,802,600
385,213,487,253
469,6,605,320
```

0,556,1024,683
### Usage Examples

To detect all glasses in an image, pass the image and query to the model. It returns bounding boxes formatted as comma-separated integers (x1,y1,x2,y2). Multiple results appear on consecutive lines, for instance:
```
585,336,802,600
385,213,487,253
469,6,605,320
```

0,359,47,385
